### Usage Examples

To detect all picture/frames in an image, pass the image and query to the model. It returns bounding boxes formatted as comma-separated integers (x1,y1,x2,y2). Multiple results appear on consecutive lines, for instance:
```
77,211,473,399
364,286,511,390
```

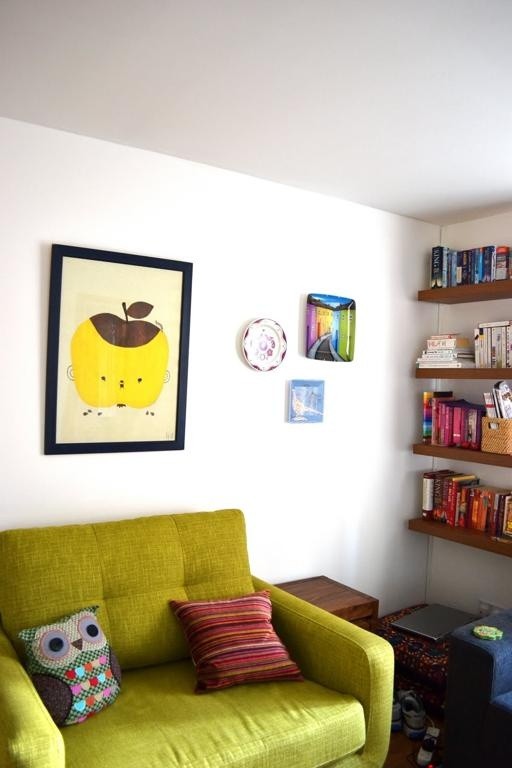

42,243,193,457
303,292,356,364
286,380,326,425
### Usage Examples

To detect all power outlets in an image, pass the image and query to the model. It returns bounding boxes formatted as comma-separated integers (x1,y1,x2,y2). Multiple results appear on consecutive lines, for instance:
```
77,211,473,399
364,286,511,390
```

477,597,503,620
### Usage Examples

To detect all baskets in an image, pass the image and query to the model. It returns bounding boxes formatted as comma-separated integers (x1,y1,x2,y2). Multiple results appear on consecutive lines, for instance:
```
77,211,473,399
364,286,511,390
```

481,416,512,455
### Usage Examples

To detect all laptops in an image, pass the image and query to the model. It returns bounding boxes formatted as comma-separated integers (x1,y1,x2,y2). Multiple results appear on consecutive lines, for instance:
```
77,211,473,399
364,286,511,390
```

388,602,480,645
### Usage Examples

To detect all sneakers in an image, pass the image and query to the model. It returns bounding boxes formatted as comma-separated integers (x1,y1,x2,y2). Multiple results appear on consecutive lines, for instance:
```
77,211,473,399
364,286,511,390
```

397,688,426,739
391,691,403,735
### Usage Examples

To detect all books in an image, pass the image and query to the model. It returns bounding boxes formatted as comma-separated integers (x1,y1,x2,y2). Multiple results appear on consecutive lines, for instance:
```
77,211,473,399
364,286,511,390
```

472,320,511,368
421,468,512,542
428,246,512,288
483,384,511,427
416,333,475,369
418,389,483,450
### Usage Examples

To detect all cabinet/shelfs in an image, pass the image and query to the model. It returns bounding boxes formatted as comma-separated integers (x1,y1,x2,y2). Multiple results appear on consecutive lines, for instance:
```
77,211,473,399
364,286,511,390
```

407,277,512,561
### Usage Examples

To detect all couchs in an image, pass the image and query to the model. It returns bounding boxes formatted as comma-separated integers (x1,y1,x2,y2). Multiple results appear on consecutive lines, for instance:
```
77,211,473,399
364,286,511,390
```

0,507,396,767
441,609,511,768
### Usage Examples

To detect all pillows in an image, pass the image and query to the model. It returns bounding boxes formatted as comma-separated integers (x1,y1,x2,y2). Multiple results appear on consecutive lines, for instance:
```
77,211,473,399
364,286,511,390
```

14,607,124,731
162,590,305,696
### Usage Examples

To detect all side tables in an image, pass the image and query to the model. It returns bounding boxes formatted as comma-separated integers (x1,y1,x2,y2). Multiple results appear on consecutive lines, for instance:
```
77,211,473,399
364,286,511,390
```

272,575,381,637
